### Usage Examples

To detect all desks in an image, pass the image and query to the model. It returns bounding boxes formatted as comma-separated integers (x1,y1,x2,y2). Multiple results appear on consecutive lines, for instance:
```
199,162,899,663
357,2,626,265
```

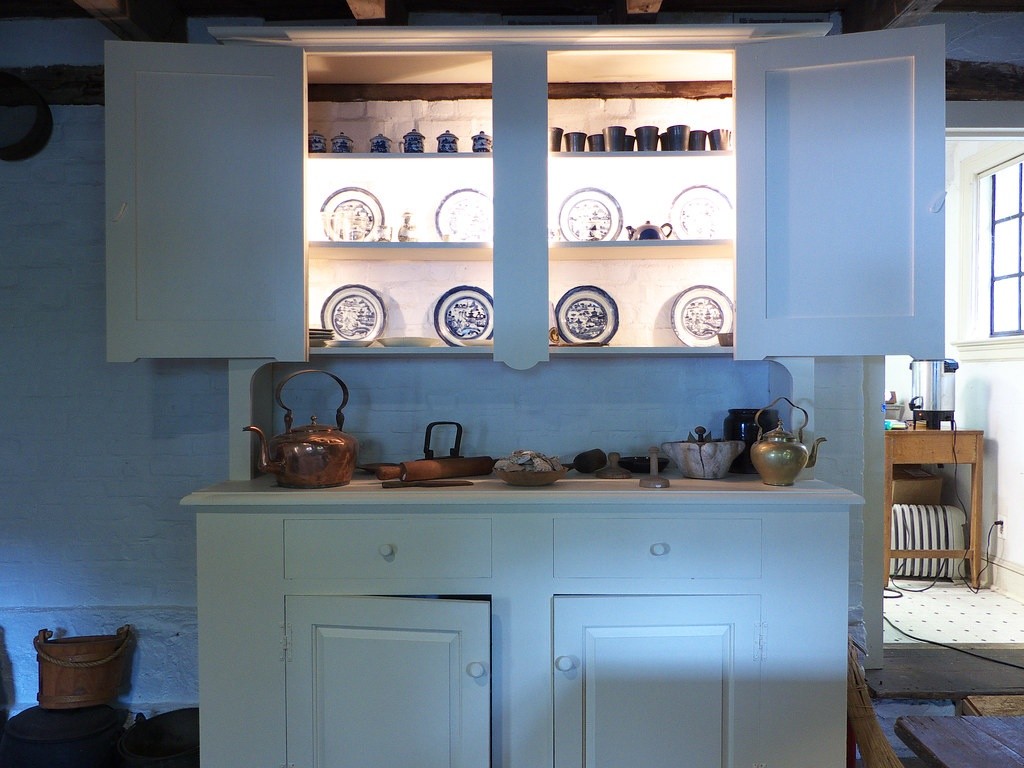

893,716,1024,768
884,430,984,588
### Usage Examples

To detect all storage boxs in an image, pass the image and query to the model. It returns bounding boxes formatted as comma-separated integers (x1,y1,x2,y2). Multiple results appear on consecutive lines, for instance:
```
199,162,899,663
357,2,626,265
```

892,464,942,506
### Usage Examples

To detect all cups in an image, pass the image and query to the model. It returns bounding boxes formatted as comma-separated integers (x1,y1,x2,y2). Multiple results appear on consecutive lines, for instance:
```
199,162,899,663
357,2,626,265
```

548,125,731,152
549,301,559,344
321,213,393,242
547,225,560,242
308,129,493,153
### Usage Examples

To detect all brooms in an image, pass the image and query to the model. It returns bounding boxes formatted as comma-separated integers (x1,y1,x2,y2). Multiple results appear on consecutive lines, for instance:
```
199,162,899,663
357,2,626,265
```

846,638,904,768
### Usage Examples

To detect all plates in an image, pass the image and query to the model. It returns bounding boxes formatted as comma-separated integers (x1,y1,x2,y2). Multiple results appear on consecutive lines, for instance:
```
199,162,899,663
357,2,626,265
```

669,185,735,239
619,457,669,472
671,284,734,347
555,285,619,344
434,285,494,347
309,284,386,347
435,188,492,242
320,187,385,242
376,337,440,347
557,187,623,241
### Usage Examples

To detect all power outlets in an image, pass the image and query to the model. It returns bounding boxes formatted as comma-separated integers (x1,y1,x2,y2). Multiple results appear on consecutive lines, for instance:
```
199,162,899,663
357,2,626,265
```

997,515,1006,539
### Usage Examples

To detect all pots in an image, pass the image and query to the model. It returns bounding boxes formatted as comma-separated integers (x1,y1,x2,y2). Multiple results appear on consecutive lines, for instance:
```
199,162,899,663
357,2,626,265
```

0,706,128,768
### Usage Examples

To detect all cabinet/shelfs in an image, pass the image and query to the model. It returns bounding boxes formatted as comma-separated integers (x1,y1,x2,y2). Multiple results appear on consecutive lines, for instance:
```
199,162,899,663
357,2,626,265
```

104,23,944,768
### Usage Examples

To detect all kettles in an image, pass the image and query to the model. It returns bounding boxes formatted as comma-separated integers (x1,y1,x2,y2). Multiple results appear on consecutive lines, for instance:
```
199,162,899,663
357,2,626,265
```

750,397,827,486
243,369,358,490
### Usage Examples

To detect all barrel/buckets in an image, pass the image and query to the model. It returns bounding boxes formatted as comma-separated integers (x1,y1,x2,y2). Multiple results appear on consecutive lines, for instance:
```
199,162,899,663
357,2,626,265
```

117,707,200,768
909,358,959,430
33,624,132,709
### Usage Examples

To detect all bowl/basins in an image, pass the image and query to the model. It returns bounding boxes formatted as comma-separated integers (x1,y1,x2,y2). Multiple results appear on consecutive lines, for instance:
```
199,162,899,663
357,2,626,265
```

716,333,733,347
493,466,568,486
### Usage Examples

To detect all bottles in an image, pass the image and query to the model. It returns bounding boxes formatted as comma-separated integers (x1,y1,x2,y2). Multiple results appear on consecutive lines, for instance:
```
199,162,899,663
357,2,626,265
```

723,409,779,474
398,212,416,242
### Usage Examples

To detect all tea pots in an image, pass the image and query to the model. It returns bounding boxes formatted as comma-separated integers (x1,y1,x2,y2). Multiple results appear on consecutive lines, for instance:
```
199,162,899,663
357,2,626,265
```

626,220,673,241
